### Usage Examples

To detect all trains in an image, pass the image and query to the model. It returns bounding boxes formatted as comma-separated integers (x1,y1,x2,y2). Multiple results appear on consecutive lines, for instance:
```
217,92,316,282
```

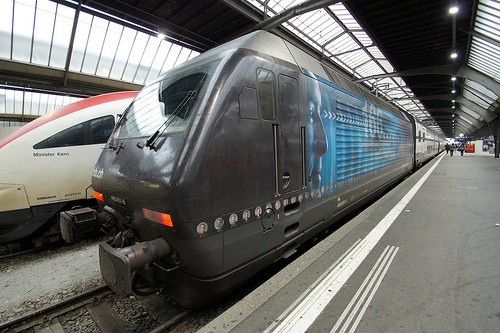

90,29,448,310
0,90,142,243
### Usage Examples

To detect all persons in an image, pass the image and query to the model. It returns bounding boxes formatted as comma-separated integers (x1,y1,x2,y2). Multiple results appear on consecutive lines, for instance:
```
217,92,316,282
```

444,142,494,156
303,71,328,189
